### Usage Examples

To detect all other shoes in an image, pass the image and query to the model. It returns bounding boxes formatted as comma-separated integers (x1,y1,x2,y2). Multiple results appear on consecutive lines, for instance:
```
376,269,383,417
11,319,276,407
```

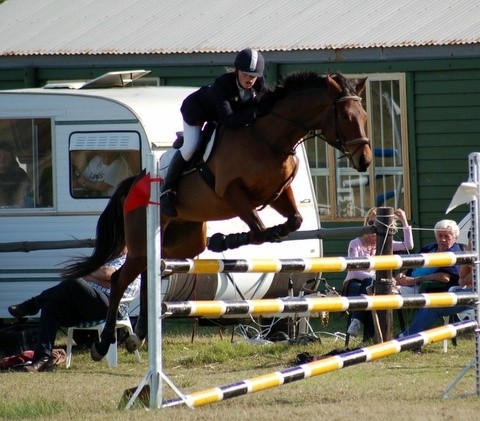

347,319,360,337
9,297,38,318
23,357,52,372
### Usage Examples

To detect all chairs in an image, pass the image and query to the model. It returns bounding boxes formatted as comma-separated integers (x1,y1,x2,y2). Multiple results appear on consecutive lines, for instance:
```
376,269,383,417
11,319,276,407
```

67,297,142,369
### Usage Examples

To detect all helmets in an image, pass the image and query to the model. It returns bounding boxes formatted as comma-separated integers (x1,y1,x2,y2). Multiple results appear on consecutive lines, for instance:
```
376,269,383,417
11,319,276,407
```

235,49,264,78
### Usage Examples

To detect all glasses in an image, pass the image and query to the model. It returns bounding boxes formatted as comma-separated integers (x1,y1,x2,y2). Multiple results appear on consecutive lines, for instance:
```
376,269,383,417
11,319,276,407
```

367,220,374,225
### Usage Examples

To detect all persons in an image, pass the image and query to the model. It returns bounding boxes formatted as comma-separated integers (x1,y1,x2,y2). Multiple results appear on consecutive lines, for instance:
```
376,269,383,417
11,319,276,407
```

343,207,414,342
162,48,270,217
77,151,129,198
8,243,141,372
396,219,476,339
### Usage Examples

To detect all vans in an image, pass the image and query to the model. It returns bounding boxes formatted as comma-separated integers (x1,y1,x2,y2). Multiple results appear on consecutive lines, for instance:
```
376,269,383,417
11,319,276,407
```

0,69,324,322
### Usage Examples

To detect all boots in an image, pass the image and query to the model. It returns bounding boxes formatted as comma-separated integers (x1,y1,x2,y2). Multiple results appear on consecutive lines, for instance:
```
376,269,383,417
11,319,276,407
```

159,151,188,217
